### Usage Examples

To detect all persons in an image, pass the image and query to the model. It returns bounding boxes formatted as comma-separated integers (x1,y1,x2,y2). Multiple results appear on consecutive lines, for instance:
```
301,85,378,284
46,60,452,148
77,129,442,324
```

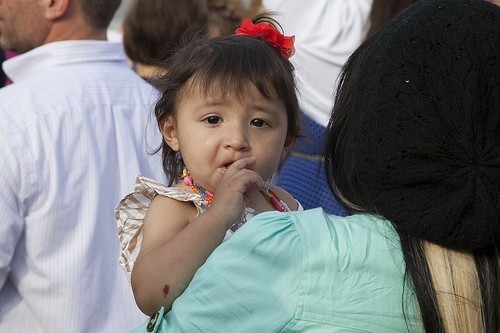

121,0,417,216
114,9,306,331
0,0,186,332
134,0,500,333
